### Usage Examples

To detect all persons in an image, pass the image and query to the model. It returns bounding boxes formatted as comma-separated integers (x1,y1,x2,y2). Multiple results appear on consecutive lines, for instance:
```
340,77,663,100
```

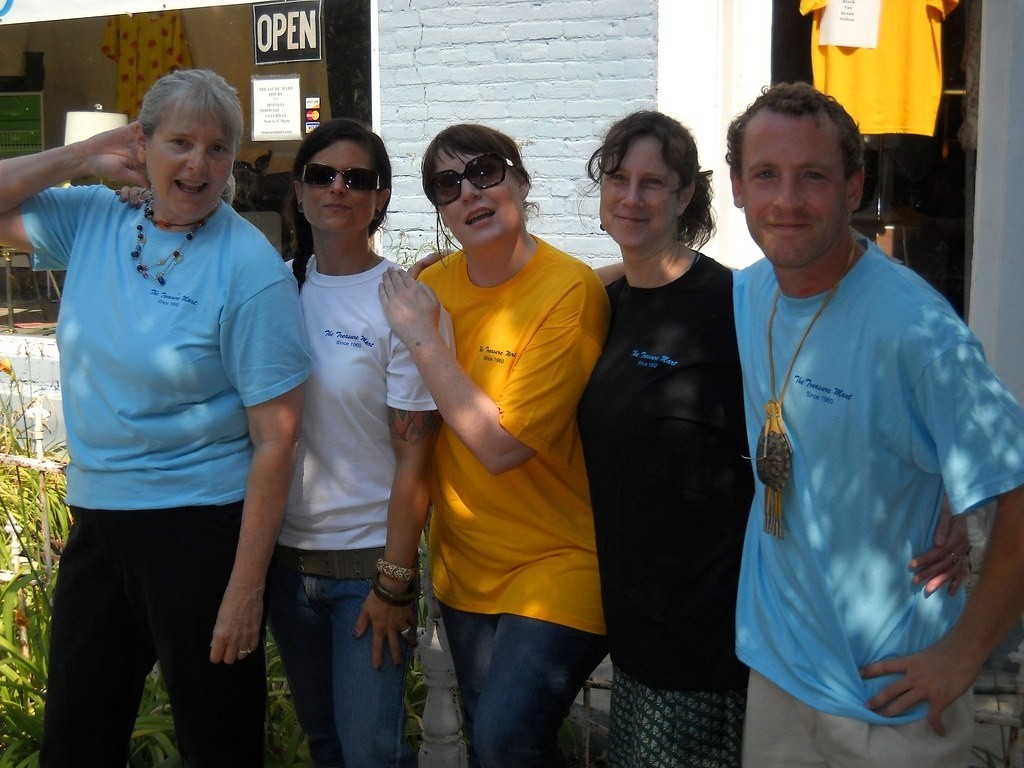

407,110,975,768
115,118,456,767
379,126,611,768
594,83,1024,768
1,70,311,768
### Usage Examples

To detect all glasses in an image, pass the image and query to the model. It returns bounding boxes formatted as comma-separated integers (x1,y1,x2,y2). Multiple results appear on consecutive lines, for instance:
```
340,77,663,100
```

425,153,515,205
300,163,386,191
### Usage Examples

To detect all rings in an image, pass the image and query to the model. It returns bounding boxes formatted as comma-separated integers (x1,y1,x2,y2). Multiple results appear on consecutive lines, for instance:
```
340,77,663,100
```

239,647,254,654
401,625,411,635
950,552,961,568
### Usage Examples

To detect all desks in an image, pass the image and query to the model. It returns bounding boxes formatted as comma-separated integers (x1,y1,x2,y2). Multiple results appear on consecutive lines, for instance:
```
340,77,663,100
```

1,247,62,330
854,218,951,269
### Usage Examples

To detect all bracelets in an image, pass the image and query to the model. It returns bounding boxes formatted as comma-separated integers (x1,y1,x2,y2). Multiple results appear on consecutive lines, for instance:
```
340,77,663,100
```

373,557,421,606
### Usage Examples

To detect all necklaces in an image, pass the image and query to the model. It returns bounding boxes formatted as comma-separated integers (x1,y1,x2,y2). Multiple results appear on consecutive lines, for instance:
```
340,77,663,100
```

130,195,219,284
755,223,857,538
